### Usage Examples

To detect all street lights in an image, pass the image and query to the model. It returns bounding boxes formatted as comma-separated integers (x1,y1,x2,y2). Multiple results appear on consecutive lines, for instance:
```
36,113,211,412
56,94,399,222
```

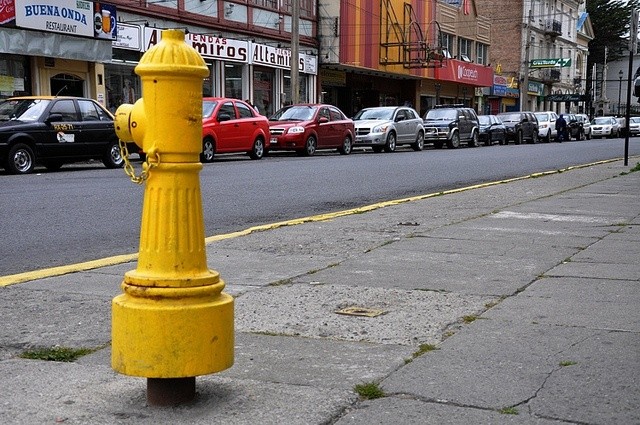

618,70,624,117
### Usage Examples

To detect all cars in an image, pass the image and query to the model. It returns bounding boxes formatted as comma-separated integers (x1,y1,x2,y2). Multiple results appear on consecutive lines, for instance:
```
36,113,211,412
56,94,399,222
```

0,95,136,175
561,114,581,141
268,104,355,156
496,112,539,144
477,115,507,146
617,117,627,138
576,113,592,141
534,111,559,142
139,97,271,162
631,117,640,127
351,106,426,152
629,116,640,137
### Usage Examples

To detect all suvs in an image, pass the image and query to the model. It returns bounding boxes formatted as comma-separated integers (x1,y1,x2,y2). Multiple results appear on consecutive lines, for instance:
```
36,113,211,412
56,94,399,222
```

423,104,479,148
591,116,620,139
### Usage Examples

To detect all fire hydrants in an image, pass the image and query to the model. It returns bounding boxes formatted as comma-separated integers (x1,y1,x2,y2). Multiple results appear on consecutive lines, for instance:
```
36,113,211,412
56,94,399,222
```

110,29,235,404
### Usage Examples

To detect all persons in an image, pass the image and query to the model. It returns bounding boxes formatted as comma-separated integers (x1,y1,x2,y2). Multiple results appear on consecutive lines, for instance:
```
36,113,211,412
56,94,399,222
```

556,113,567,143
